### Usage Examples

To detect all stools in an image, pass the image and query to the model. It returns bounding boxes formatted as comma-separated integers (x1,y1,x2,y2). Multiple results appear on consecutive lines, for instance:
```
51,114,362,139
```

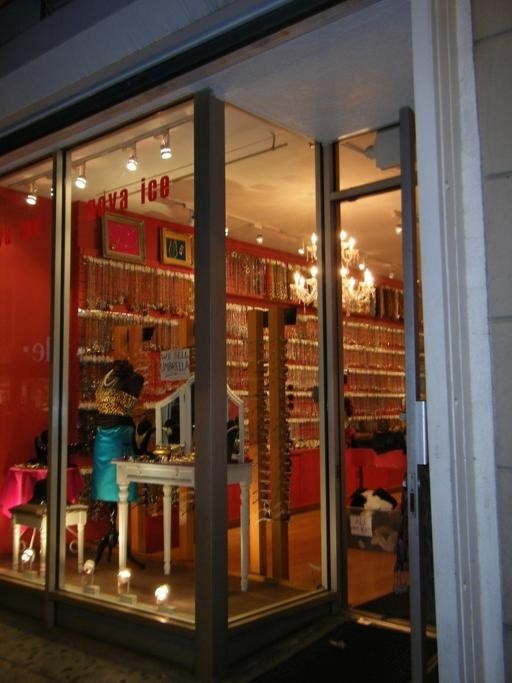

8,499,90,578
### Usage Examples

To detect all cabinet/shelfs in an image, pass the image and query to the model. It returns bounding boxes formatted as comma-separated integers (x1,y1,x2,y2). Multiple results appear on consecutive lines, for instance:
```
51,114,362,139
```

346,444,377,502
141,501,183,555
224,474,245,532
304,449,322,514
376,444,410,495
106,372,253,598
285,446,302,518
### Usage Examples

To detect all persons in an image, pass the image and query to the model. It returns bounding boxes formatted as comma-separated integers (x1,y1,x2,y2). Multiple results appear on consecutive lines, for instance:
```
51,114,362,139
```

93,359,144,533
177,243,185,259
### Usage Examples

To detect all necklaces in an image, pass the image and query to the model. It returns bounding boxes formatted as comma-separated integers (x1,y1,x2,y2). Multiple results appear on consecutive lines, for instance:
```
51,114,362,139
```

77,252,193,412
343,320,407,434
38,433,49,451
226,252,321,449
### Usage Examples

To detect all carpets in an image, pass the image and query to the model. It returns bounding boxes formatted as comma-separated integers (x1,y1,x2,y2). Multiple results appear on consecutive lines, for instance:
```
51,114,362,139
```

250,609,438,682
352,583,436,626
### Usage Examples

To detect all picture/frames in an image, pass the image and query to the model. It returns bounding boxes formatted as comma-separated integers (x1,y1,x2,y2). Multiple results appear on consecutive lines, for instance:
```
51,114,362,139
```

157,225,197,271
101,208,146,267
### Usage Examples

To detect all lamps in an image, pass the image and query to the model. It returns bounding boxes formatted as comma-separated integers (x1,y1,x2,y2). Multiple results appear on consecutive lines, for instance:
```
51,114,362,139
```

292,220,378,319
5,112,199,207
